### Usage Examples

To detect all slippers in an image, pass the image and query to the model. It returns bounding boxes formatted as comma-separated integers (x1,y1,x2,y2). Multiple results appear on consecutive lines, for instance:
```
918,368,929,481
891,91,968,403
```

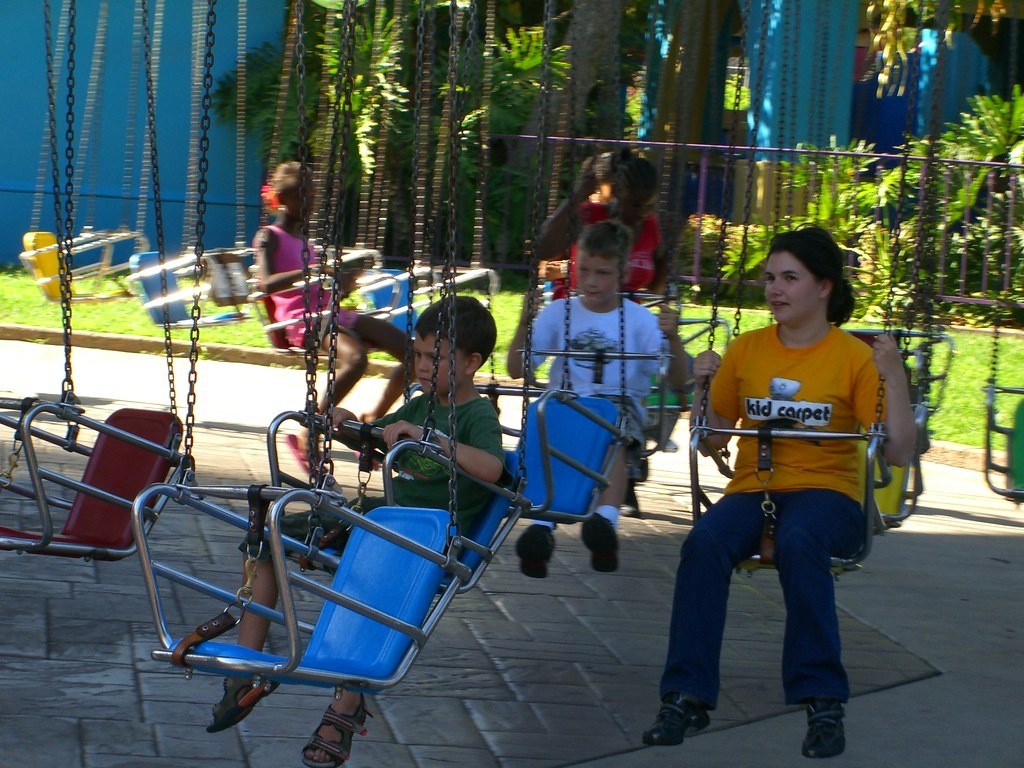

355,413,379,470
286,433,328,481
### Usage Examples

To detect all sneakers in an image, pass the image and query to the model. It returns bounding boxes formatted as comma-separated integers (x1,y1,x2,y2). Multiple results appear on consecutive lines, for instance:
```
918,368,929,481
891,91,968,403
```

802,700,844,759
641,691,710,746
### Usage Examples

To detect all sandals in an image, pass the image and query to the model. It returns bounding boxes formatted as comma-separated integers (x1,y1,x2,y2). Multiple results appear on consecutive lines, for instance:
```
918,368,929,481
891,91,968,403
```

301,691,373,768
206,673,280,732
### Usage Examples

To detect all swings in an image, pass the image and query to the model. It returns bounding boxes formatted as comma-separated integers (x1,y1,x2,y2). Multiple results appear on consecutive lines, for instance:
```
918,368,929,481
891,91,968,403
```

0,0,1024,714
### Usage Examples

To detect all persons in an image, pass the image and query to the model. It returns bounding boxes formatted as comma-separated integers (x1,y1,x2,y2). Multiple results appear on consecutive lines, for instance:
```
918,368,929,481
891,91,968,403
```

253,162,417,482
643,223,920,760
207,296,514,768
507,220,685,579
535,147,672,304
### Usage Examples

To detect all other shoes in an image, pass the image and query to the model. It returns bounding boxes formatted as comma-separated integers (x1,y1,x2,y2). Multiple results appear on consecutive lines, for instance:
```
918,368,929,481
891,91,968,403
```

515,525,555,579
582,514,619,572
638,458,648,482
657,439,678,452
619,492,640,518
